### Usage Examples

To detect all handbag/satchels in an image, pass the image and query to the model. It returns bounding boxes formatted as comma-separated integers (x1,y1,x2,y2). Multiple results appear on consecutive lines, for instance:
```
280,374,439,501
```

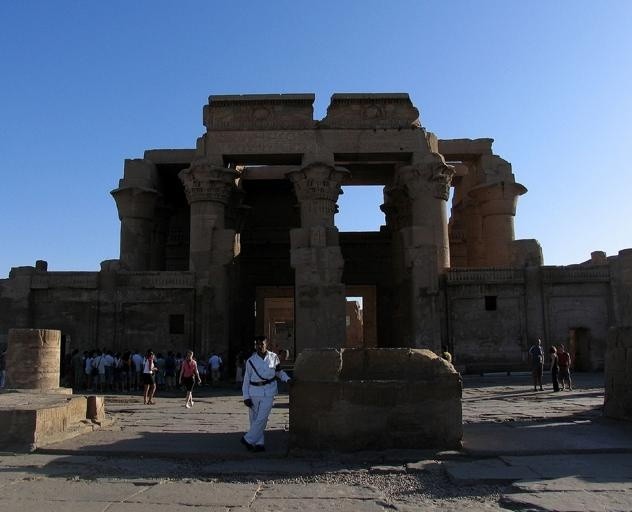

92,368,99,376
113,368,122,374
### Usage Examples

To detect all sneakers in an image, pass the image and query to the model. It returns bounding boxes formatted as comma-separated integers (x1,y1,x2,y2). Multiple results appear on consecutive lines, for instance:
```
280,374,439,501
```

185,401,194,409
241,436,265,452
75,387,142,395
144,401,156,405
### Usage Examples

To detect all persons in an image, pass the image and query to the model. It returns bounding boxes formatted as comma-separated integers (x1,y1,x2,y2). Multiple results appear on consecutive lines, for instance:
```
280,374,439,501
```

241,337,293,452
0,342,9,389
61,345,246,409
438,343,452,362
528,338,573,392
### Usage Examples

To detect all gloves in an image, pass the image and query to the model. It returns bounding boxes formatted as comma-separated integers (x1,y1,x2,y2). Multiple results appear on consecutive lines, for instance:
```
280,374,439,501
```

244,399,254,409
288,380,296,386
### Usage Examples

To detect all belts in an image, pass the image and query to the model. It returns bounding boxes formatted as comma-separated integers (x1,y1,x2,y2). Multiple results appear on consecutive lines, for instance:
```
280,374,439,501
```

250,376,276,386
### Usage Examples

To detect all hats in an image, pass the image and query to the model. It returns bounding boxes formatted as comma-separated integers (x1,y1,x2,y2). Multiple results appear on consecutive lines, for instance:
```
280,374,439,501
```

148,352,155,355
